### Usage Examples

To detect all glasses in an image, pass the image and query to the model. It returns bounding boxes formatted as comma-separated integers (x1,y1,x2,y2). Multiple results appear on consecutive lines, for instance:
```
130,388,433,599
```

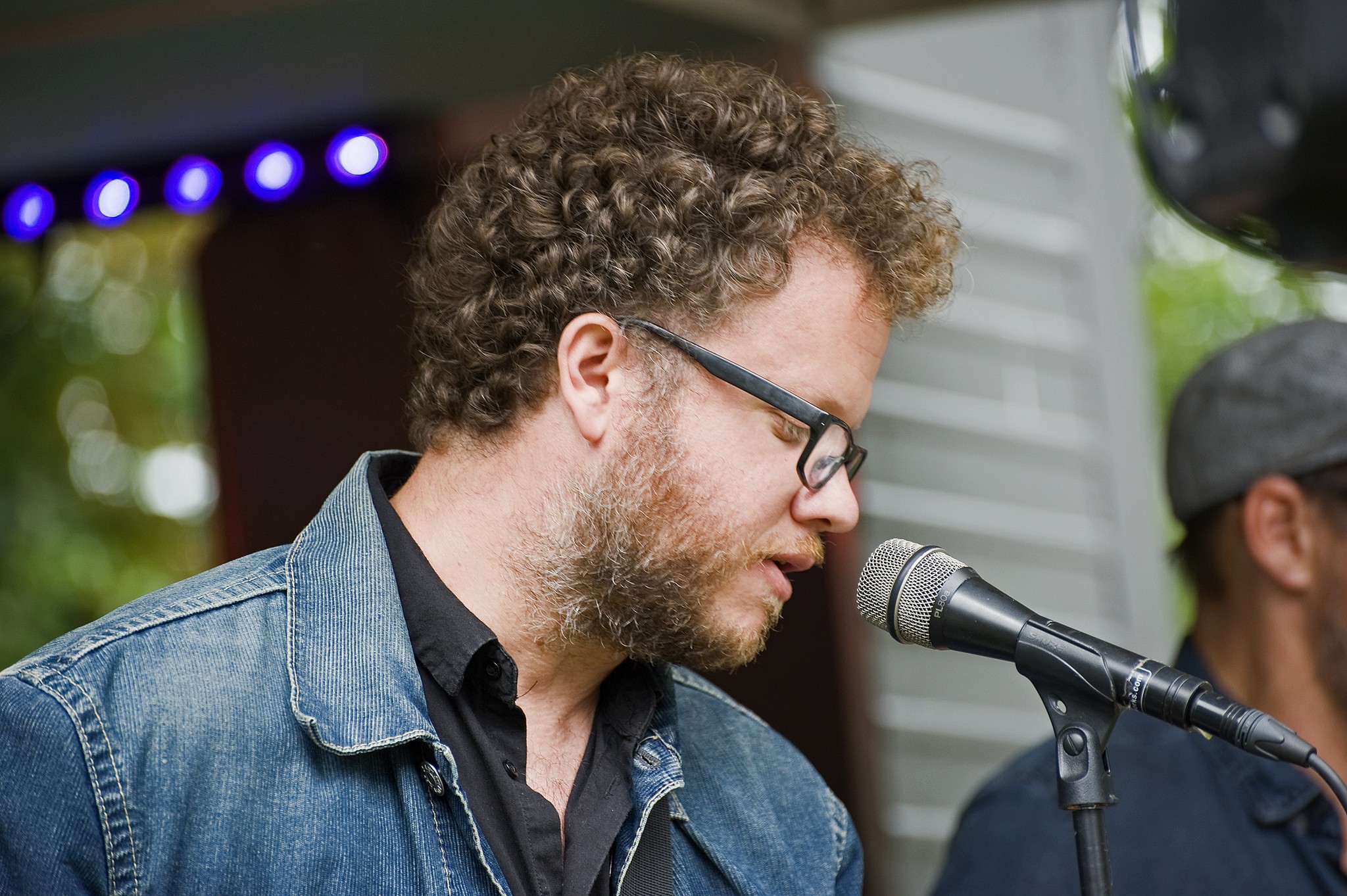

610,316,870,492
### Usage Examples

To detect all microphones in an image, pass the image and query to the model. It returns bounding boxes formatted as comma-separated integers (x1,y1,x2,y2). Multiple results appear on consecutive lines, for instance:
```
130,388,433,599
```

855,537,1318,768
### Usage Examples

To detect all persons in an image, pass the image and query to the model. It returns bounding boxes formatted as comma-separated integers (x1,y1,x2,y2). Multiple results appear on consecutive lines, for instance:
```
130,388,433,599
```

930,320,1346,896
0,57,953,896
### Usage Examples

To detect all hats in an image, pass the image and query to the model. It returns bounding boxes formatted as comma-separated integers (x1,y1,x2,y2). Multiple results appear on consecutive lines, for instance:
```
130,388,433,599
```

1164,317,1347,520
